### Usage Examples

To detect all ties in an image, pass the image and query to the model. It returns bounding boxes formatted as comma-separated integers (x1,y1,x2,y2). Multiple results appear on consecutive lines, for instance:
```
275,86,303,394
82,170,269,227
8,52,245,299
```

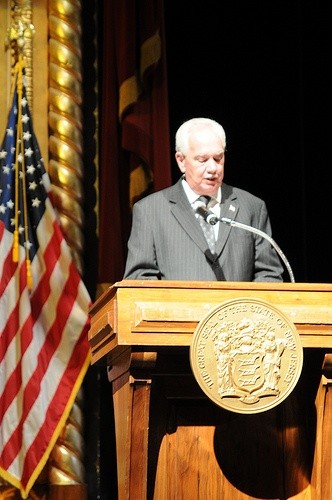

192,196,215,254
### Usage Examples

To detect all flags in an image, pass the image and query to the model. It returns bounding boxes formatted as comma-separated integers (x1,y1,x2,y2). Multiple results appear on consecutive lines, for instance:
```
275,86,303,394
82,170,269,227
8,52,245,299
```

0,60,97,500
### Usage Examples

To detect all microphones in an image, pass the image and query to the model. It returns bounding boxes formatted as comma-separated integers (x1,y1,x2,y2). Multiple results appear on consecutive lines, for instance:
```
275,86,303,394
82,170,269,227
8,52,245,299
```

193,201,218,226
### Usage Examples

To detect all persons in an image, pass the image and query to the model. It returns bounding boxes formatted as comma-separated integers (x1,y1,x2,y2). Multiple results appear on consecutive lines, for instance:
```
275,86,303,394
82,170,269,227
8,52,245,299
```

122,118,286,283
